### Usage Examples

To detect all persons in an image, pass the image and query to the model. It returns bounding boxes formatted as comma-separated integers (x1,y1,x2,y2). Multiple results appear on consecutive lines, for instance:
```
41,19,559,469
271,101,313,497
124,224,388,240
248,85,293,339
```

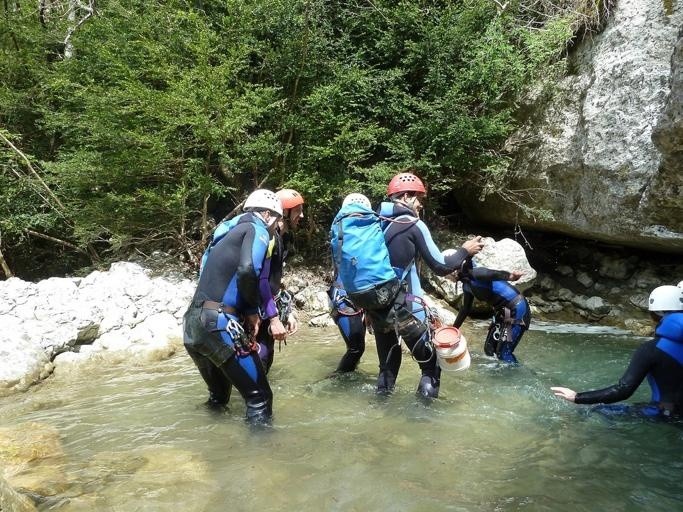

550,285,683,430
257,189,305,375
182,189,287,430
327,191,373,379
441,248,532,367
365,172,483,396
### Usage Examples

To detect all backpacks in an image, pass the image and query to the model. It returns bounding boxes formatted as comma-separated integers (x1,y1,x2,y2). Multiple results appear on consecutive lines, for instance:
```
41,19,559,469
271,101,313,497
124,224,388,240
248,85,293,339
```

328,202,417,315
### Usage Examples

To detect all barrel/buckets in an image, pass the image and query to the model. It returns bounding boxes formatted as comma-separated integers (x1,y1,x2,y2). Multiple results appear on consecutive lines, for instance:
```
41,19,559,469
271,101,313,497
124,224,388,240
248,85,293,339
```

432,326,471,377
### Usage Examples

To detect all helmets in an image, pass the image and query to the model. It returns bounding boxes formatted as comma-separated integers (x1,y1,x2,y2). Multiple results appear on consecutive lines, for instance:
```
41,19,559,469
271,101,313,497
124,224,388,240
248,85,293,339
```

648,285,683,311
388,173,427,198
441,249,457,256
243,189,283,217
342,193,371,210
276,189,304,213
677,281,683,292
